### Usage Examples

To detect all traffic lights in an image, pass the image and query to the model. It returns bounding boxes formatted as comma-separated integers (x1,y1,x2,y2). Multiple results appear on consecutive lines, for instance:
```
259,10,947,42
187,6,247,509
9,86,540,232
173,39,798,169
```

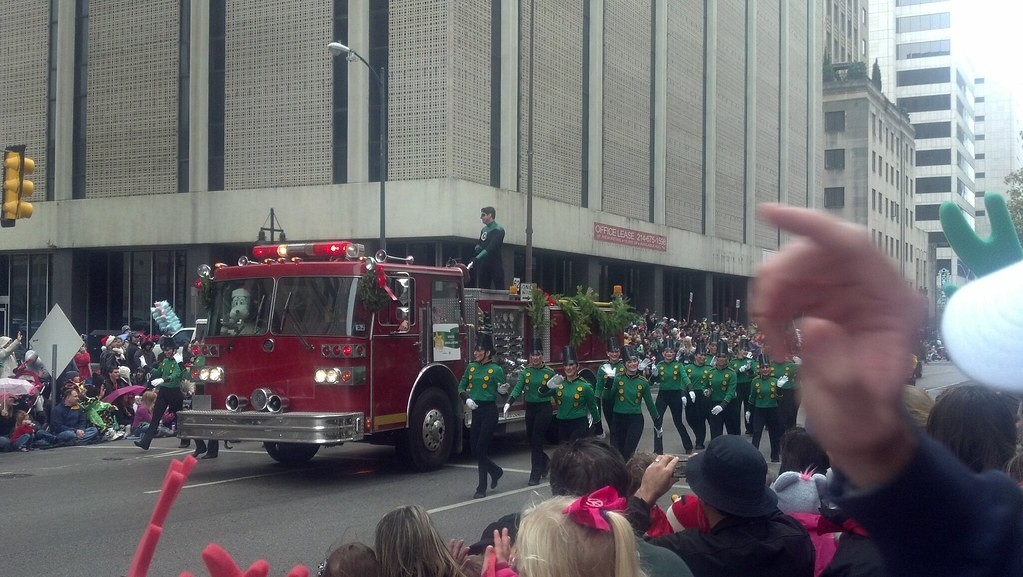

1,144,35,227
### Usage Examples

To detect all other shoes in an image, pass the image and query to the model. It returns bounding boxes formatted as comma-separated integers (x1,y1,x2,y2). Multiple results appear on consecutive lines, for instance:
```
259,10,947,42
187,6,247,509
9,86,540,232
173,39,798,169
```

133,440,149,450
684,446,693,456
473,467,503,498
193,448,217,459
178,439,190,448
528,459,551,486
695,443,705,449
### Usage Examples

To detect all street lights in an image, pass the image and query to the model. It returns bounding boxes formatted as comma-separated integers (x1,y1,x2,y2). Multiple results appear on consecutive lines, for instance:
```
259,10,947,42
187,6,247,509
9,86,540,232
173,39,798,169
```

327,42,386,253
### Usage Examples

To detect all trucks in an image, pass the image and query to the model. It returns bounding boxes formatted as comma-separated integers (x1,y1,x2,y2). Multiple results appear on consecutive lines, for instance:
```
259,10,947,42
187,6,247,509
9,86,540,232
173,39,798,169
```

139,319,243,359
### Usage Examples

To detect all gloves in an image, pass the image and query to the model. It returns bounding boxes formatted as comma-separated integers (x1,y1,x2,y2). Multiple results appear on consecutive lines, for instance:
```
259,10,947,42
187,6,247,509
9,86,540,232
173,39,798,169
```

503,403,511,420
655,426,664,438
466,398,479,411
636,358,651,372
151,378,166,386
140,355,147,369
651,363,659,377
745,411,750,423
777,375,788,388
496,382,510,395
739,365,748,372
601,362,616,377
547,374,564,389
174,353,183,364
681,388,723,415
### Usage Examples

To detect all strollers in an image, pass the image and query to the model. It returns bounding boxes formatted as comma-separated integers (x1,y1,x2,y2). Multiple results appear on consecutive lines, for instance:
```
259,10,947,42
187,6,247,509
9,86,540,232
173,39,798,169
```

0,370,49,435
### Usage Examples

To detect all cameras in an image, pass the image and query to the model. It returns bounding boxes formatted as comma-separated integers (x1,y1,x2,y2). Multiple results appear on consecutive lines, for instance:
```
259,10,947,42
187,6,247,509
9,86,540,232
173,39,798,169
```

673,461,689,478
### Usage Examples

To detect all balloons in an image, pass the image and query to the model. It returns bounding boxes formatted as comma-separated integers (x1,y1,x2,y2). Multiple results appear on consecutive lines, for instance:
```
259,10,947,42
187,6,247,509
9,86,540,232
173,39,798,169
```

150,300,183,335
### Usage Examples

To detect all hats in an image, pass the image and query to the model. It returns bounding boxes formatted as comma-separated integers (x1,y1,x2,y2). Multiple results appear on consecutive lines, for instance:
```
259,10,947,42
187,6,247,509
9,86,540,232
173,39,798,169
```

101,324,179,351
66,371,79,379
685,435,778,516
770,471,828,516
475,330,772,369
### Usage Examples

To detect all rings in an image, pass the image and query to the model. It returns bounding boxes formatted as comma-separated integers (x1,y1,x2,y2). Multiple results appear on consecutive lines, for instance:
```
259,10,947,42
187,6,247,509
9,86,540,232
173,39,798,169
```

744,296,781,320
653,459,660,462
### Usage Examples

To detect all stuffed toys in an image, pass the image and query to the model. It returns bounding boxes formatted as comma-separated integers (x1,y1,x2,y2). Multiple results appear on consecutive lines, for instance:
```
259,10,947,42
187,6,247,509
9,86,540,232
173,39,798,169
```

220,288,262,335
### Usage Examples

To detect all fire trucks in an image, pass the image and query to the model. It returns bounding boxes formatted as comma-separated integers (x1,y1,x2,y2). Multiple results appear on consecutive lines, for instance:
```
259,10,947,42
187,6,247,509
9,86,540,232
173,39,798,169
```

175,243,625,473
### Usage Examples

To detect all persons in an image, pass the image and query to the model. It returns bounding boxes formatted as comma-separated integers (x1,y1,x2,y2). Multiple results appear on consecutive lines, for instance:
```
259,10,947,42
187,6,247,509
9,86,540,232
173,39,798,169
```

0,325,243,459
319,382,1023,577
457,306,950,505
467,207,505,290
751,199,1023,577
352,276,408,330
